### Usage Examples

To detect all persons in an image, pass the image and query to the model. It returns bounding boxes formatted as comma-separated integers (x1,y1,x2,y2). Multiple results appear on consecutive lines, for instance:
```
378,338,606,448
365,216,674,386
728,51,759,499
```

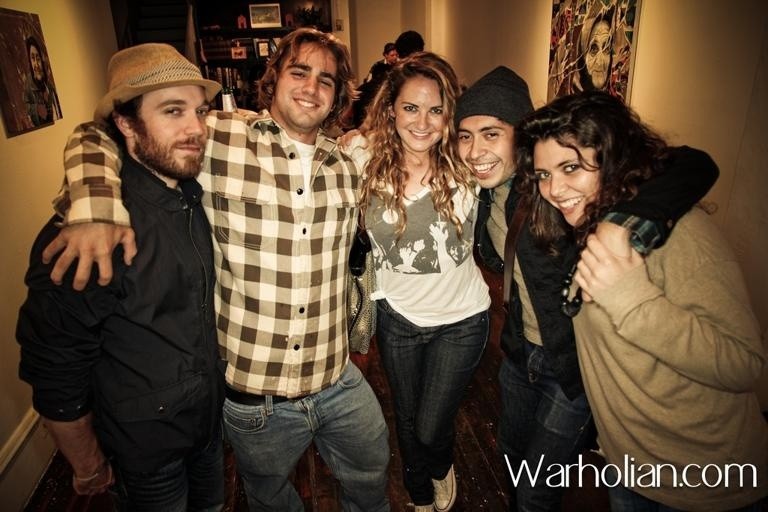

361,44,398,84
20,36,63,124
515,92,768,512
338,63,718,511
339,52,494,512
42,28,392,510
567,12,623,95
15,42,232,512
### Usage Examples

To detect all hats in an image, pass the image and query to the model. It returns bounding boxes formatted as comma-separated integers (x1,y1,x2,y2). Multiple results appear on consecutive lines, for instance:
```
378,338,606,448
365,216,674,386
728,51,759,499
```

452,66,535,135
93,43,223,117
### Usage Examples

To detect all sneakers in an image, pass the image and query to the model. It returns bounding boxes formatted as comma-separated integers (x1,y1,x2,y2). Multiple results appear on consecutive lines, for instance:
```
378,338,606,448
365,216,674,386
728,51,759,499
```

431,463,458,512
415,506,434,512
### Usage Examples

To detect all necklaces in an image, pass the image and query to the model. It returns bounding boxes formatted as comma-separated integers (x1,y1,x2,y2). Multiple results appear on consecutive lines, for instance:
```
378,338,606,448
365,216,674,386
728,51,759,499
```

556,219,610,320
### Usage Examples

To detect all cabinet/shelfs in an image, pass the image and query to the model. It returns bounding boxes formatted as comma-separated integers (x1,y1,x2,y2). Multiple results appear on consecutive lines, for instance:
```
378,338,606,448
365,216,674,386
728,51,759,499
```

188,0,339,109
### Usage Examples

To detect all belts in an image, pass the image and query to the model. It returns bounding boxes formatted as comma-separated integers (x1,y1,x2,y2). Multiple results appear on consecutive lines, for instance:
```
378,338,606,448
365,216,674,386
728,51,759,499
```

222,385,313,404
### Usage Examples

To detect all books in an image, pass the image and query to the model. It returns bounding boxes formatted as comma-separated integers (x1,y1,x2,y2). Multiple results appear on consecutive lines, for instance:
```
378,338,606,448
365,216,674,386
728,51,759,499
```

198,66,248,92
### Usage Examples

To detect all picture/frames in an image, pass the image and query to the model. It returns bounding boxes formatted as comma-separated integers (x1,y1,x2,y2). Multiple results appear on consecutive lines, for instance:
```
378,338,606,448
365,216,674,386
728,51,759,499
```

546,1,644,115
0,7,65,139
